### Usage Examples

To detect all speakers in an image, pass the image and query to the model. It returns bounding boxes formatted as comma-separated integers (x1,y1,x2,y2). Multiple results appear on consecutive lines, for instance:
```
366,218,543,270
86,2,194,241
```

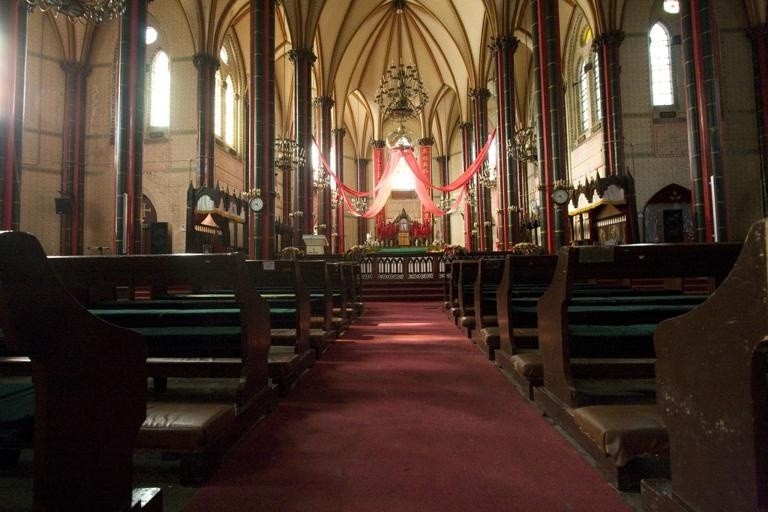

149,222,172,252
56,198,65,213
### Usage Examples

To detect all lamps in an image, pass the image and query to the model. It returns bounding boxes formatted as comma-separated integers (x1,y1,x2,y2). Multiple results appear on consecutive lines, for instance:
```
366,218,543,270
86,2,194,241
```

437,14,537,212
275,7,365,216
374,0,429,120
24,0,127,27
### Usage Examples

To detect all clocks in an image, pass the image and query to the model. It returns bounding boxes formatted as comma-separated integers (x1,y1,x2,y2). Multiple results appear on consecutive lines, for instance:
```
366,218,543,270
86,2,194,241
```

550,188,569,205
249,196,265,213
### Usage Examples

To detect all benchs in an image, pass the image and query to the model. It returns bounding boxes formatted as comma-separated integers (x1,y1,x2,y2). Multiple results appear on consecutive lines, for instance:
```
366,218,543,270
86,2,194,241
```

444,219,768,512
0,230,363,512
